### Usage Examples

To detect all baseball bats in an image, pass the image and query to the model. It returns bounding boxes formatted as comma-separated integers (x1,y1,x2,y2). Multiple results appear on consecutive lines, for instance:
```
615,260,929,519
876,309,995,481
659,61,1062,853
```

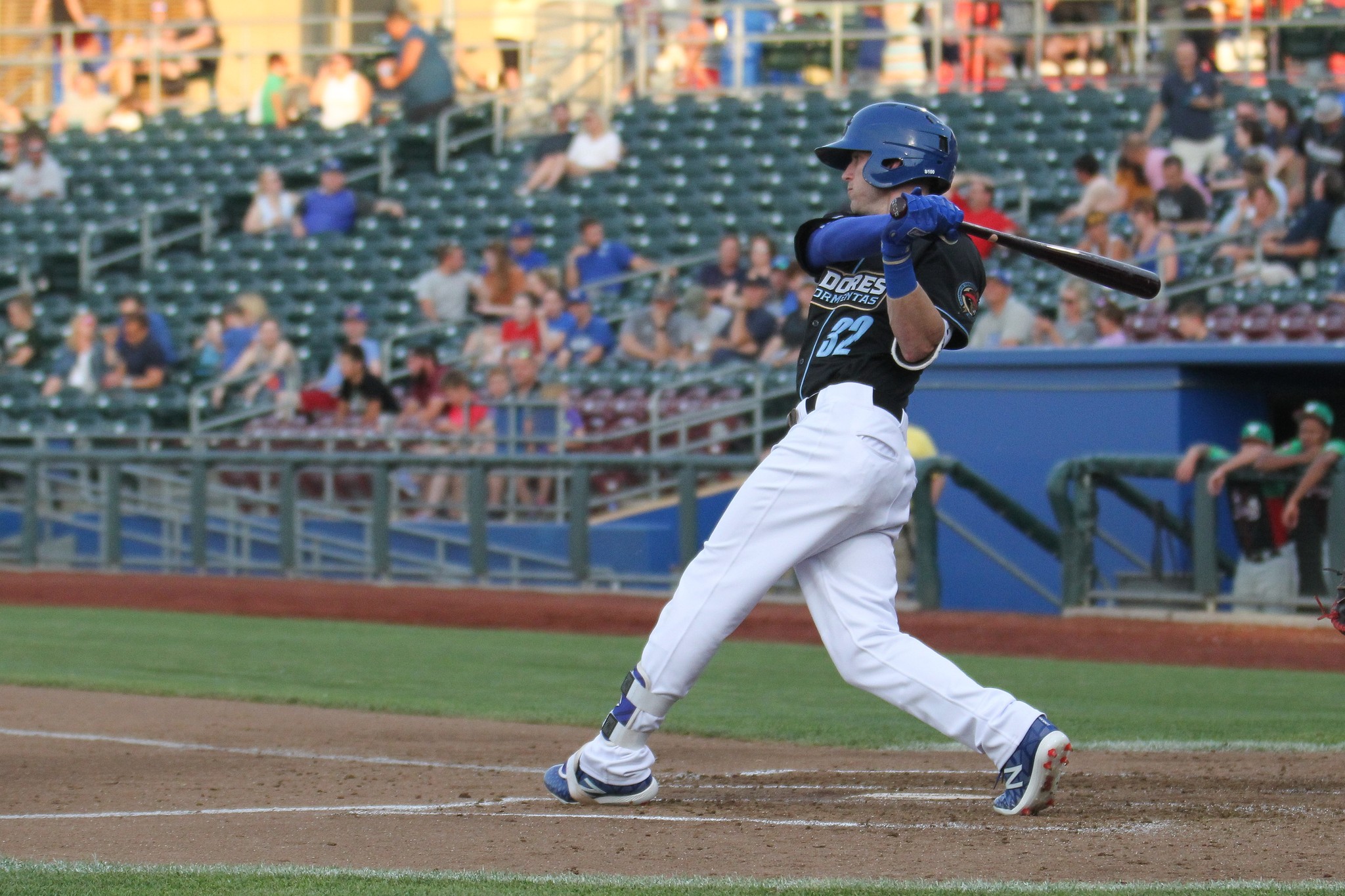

892,193,1165,300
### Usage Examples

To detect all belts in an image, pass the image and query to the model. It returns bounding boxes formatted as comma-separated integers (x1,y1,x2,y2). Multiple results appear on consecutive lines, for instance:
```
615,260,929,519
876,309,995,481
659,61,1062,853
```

1244,546,1280,564
784,382,903,427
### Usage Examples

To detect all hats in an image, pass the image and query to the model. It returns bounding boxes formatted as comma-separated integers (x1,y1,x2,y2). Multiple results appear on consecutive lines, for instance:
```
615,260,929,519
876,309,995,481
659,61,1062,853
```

317,158,342,174
1294,401,1333,429
339,302,366,321
1242,422,1272,444
566,289,587,303
506,219,529,237
651,283,678,303
741,275,771,289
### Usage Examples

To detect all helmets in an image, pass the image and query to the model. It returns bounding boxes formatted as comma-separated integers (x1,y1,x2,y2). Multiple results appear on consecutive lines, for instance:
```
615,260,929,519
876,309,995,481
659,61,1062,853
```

815,101,958,198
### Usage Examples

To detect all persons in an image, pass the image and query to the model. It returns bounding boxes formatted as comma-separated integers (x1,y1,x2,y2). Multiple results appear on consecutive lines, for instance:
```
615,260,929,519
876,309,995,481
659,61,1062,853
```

541,102,1070,816
1175,400,1345,616
946,40,1345,348
0,13,818,522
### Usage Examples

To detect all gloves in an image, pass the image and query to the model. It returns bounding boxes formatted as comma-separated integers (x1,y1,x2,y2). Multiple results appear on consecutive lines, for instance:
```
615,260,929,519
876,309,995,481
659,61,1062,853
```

880,186,963,298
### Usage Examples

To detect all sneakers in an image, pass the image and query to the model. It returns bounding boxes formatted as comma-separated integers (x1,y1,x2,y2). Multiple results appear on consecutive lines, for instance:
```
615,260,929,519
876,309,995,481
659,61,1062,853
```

542,757,657,809
992,717,1074,816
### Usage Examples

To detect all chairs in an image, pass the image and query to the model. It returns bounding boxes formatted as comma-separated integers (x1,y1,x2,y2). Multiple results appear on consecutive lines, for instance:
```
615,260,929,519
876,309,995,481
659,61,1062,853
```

0,80,1156,462
